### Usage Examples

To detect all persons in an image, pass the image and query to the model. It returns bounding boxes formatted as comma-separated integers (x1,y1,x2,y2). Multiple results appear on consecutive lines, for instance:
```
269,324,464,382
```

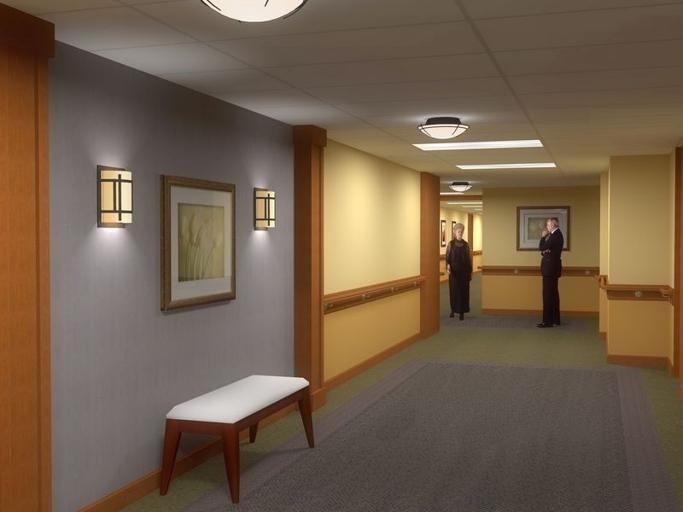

534,216,564,328
445,222,473,321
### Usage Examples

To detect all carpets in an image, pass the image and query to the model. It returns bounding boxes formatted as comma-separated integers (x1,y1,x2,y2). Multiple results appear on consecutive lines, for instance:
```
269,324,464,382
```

188,358,679,512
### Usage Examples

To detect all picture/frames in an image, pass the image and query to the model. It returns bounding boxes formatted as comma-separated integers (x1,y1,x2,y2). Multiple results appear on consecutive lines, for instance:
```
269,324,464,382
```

516,205,570,251
159,173,236,311
452,221,456,239
441,220,446,247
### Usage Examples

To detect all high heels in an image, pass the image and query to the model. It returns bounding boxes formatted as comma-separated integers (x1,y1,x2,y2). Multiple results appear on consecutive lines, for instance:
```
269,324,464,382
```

449,311,464,320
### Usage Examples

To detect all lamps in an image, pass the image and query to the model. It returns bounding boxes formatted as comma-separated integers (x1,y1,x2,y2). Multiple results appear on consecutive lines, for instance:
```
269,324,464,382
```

417,117,469,140
96,164,132,228
448,182,472,192
253,187,277,230
201,0,308,23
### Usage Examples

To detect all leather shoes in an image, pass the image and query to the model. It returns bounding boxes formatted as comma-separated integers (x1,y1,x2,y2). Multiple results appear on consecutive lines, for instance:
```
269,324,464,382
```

536,320,561,328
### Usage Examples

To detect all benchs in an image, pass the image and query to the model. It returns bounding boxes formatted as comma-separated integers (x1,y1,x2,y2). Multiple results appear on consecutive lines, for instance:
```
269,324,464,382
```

157,373,315,504
